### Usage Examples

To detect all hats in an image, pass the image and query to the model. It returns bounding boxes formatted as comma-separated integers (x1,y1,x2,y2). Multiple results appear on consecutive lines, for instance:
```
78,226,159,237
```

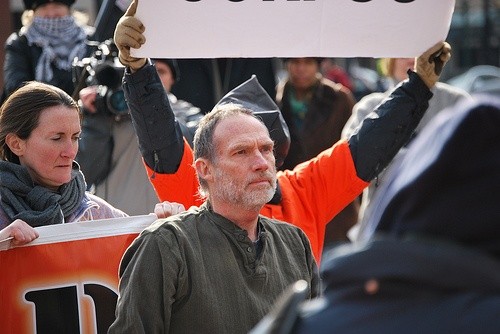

22,0,76,11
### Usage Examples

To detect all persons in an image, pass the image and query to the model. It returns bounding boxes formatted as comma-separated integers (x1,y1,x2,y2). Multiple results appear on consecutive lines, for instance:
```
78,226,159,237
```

262,57,354,248
0,83,130,253
106,104,321,334
341,57,470,237
87,59,207,218
113,1,453,261
0,1,94,98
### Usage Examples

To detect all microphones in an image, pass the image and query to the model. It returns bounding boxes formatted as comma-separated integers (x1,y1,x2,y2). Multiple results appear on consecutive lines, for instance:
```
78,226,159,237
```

94,64,120,89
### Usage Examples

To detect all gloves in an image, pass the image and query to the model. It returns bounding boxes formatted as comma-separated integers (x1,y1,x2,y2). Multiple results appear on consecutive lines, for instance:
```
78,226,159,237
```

414,40,451,89
114,0,146,70
